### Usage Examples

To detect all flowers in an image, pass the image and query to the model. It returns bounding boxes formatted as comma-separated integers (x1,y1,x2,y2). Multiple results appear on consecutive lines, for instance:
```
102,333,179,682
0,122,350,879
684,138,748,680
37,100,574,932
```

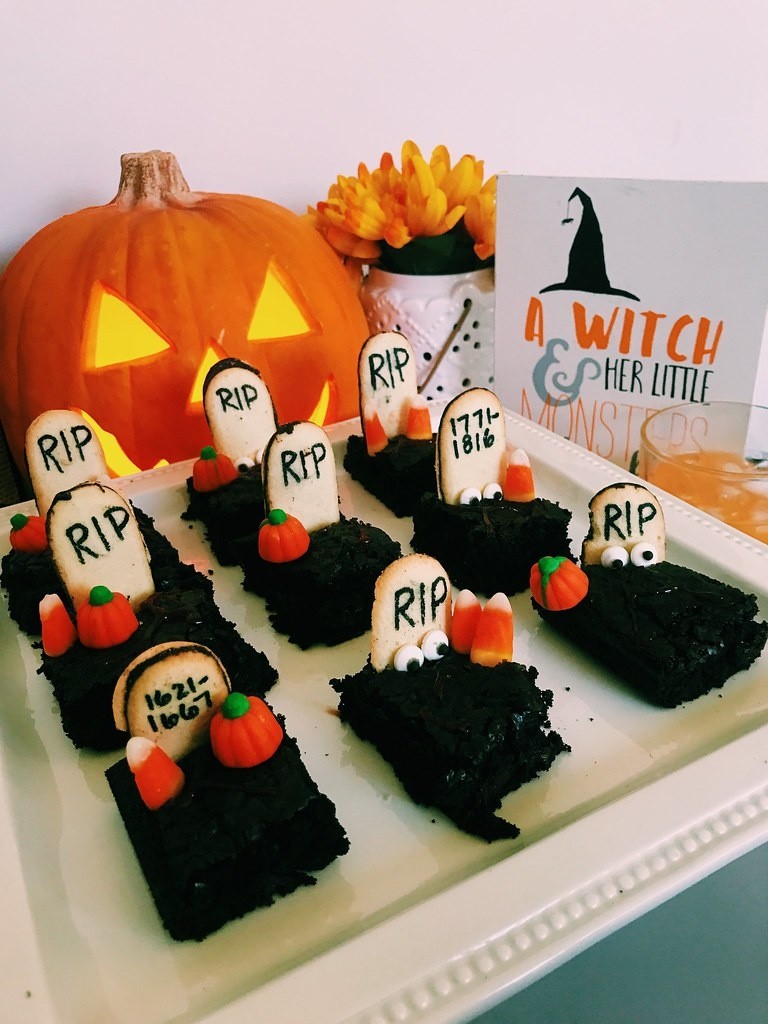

309,139,500,275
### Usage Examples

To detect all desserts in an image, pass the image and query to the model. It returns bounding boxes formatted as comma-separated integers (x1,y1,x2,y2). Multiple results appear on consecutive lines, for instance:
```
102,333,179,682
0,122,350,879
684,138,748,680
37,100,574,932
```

0,328,768,943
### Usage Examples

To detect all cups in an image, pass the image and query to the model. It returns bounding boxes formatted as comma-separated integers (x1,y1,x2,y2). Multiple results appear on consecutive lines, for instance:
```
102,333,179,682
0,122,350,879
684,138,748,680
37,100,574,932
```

639,400,768,546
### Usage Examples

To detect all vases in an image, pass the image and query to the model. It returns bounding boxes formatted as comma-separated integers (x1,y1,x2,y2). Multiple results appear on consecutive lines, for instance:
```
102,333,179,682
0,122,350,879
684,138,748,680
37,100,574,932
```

355,266,498,402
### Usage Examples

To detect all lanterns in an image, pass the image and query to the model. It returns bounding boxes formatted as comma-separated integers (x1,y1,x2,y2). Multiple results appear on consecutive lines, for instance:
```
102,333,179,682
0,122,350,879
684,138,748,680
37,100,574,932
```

0,150,372,480
